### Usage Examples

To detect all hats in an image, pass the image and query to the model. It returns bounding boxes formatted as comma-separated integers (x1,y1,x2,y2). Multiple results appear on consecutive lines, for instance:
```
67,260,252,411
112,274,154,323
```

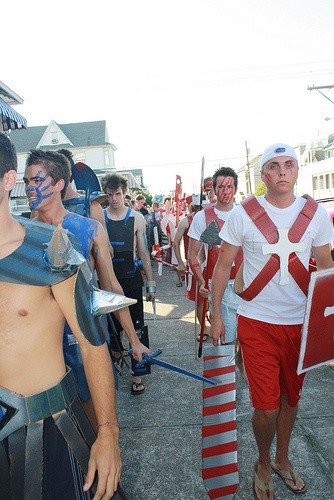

260,142,298,170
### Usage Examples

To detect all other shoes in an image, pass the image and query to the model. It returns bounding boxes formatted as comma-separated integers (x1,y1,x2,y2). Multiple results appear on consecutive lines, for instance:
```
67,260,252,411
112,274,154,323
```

181,276,185,281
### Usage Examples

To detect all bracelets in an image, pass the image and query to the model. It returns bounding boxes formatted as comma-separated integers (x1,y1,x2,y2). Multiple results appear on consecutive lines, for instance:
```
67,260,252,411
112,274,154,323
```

95,422,120,431
146,287,156,293
146,281,156,287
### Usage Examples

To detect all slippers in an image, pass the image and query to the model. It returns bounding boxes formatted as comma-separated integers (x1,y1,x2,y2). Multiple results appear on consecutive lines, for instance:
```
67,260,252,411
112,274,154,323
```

271,466,308,494
177,283,182,287
206,311,211,324
131,380,145,395
253,462,275,500
197,333,208,342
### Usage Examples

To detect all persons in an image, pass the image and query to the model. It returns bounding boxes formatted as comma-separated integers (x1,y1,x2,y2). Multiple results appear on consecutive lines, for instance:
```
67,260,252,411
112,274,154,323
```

18,149,156,436
0,132,127,500
125,194,192,287
210,143,334,500
172,167,246,373
